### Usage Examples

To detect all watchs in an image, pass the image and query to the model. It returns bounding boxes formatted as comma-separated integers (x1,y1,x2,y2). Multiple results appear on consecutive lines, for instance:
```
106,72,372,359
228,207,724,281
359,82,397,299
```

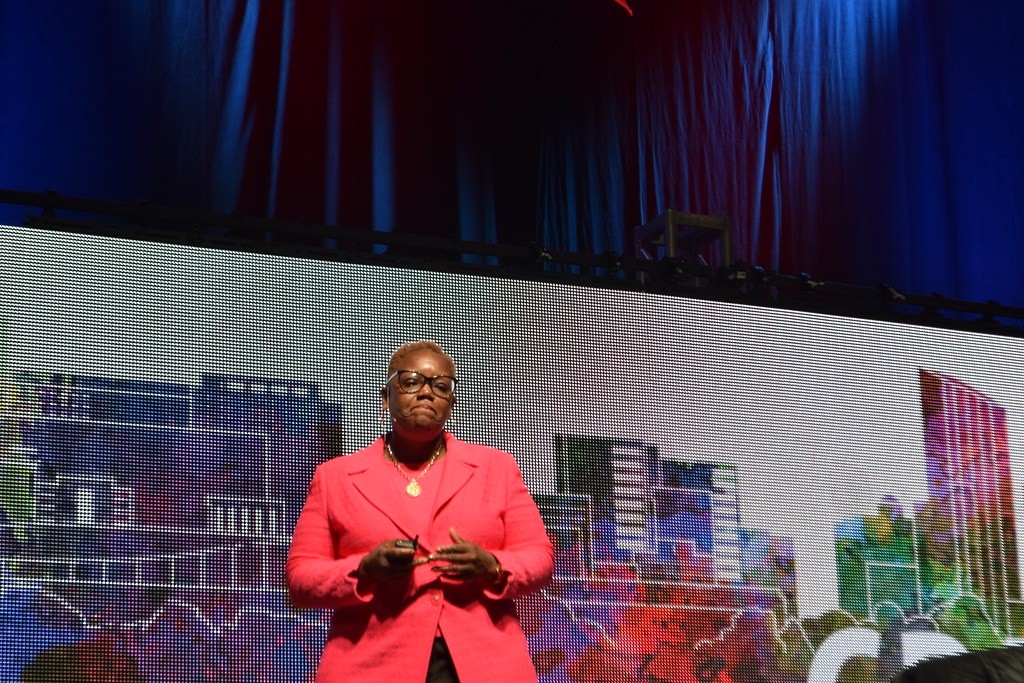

492,563,506,586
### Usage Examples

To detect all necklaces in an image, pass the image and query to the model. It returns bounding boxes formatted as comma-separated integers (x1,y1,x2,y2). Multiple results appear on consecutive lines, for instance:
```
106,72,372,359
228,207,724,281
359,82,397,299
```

386,441,443,497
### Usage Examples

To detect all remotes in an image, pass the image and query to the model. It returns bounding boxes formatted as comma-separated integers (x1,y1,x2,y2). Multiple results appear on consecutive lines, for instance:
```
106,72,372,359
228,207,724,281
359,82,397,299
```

394,534,419,551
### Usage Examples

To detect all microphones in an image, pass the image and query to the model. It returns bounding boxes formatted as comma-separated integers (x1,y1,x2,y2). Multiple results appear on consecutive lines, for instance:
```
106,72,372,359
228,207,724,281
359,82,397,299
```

387,401,396,421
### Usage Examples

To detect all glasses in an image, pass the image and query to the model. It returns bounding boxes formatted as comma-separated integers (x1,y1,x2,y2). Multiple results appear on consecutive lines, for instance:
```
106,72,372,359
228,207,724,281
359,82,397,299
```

385,369,457,399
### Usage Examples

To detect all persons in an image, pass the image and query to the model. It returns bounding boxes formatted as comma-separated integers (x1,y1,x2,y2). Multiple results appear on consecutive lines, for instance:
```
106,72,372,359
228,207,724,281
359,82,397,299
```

285,341,554,683
